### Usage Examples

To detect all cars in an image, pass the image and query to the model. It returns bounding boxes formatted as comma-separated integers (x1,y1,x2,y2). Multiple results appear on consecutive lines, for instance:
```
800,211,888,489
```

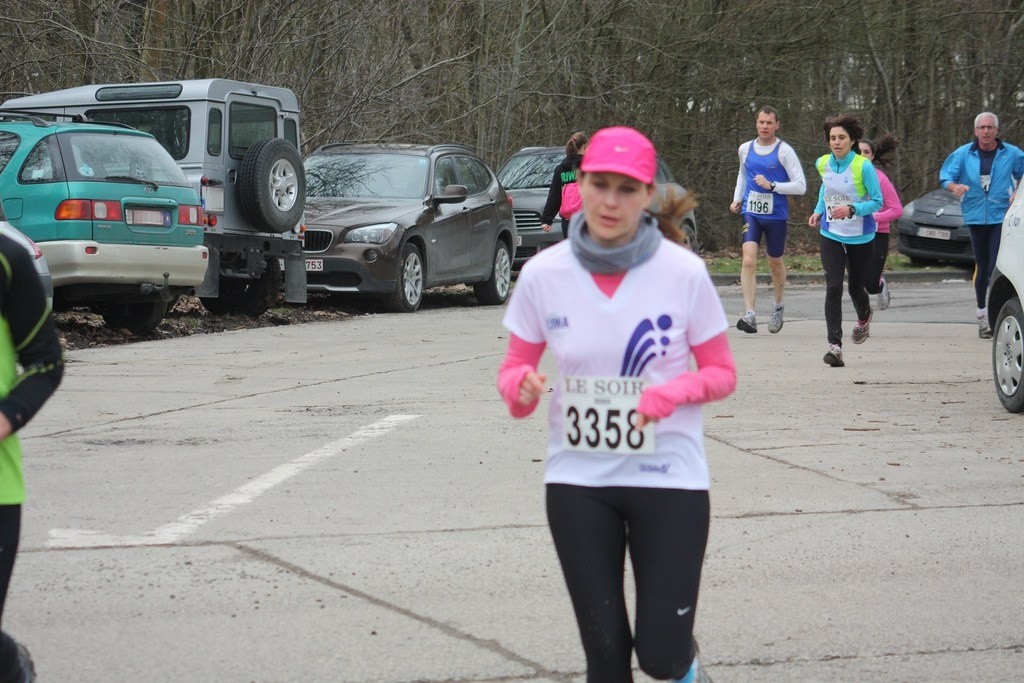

985,171,1024,414
896,184,976,267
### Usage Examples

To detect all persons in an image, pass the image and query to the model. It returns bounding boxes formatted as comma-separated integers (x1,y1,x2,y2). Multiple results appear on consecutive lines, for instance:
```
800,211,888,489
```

809,113,883,367
730,106,807,333
938,112,1024,339
859,134,904,311
497,126,737,683
541,132,589,239
0,201,65,683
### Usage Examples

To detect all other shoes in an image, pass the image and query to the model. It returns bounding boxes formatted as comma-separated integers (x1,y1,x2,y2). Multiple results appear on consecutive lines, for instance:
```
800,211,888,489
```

11,642,37,683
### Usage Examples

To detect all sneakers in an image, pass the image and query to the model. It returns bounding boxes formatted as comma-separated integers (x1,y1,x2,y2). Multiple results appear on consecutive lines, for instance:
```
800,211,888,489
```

852,307,873,344
876,278,890,310
977,312,993,338
823,343,845,367
671,638,712,683
767,298,784,333
736,311,757,333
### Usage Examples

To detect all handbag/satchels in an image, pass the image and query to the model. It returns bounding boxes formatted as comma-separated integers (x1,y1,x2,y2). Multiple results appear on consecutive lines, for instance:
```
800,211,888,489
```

558,182,583,220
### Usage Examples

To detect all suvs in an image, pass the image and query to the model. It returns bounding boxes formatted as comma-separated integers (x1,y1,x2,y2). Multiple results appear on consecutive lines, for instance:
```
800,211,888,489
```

303,141,517,312
496,146,698,272
0,108,209,332
0,78,307,319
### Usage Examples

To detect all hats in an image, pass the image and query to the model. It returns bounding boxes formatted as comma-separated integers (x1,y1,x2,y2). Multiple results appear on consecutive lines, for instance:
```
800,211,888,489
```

583,127,656,184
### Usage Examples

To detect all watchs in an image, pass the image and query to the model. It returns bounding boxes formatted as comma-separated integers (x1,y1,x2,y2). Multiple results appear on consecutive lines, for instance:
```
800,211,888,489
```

770,182,776,191
850,207,855,214
